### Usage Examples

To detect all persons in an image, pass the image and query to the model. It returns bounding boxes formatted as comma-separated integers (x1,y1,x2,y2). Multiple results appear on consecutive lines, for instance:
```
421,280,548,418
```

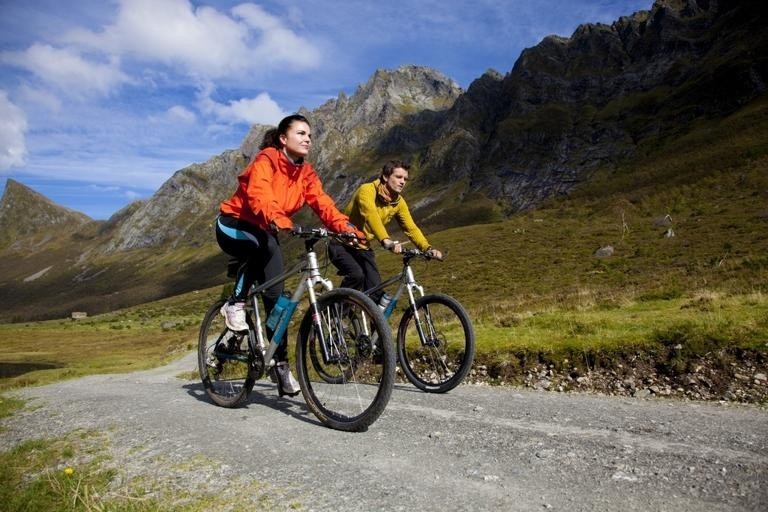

328,160,443,365
215,115,367,395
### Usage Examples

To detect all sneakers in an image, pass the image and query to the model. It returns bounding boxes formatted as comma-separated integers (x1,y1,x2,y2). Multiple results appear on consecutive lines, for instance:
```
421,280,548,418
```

372,351,402,365
219,301,250,335
268,363,302,395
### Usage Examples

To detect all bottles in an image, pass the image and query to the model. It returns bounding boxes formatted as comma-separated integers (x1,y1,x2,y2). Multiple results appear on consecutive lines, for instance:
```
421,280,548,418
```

265,291,292,333
374,293,392,312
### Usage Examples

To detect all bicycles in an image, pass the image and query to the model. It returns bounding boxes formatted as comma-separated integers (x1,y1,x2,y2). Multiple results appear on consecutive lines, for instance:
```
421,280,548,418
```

308,240,475,394
197,226,396,432
